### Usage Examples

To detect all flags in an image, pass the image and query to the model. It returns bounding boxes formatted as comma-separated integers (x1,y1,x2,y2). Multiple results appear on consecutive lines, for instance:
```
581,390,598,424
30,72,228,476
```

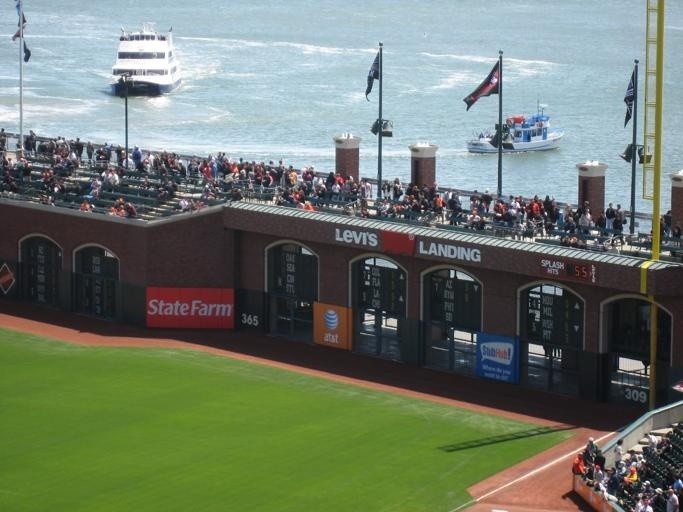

461,60,500,111
364,52,380,102
621,64,635,130
10,0,31,64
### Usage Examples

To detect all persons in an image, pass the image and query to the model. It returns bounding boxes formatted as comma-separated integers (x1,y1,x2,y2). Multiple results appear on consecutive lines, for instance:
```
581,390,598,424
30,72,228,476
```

181,151,682,264
569,431,683,512
1,127,186,218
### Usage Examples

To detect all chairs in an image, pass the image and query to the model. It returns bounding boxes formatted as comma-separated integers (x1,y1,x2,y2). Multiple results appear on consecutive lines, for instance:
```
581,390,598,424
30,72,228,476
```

607,421,683,512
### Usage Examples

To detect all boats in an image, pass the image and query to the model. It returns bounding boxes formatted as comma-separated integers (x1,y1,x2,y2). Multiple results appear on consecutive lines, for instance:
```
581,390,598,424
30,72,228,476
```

467,103,564,153
110,24,182,95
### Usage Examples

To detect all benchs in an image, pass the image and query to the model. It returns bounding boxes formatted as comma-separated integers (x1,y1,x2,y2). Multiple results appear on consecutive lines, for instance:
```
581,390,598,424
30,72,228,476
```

0,132,683,258
